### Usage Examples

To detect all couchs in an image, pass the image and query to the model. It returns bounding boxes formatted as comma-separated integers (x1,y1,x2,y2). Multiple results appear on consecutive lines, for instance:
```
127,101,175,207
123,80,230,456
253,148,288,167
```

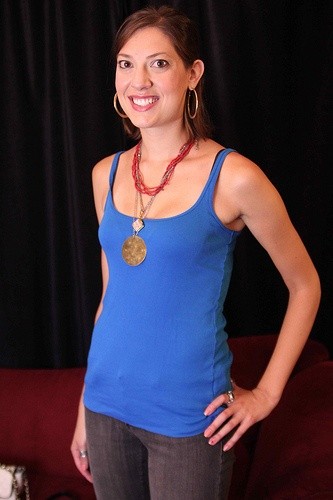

0,335,333,500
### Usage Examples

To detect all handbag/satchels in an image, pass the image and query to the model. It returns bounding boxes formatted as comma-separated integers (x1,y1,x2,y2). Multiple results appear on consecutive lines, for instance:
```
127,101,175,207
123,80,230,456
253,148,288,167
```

0,465,30,500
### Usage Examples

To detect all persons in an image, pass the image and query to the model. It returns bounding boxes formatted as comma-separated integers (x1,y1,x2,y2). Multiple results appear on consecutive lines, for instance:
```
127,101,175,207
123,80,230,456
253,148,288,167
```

71,7,322,500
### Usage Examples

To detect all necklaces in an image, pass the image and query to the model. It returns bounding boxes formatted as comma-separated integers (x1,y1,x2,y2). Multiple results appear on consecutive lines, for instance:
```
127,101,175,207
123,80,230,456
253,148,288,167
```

121,121,198,266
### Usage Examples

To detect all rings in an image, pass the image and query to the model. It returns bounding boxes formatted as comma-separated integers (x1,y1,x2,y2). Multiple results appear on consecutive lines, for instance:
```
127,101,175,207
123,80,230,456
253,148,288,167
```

225,390,235,404
79,450,88,458
